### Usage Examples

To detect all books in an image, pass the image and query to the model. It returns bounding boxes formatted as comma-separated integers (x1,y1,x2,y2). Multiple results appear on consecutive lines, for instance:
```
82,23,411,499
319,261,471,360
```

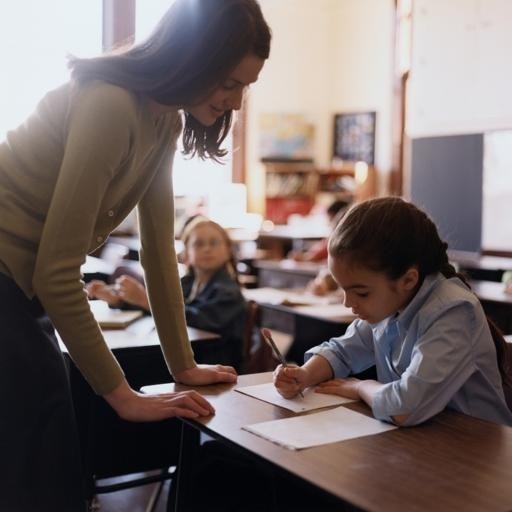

93,310,145,330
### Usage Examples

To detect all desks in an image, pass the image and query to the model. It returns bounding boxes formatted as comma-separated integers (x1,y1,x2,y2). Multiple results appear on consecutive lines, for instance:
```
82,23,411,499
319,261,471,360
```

54,219,512,512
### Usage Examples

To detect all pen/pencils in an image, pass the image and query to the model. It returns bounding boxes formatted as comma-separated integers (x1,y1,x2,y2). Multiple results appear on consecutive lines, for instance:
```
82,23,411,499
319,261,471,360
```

99,286,118,291
262,329,306,400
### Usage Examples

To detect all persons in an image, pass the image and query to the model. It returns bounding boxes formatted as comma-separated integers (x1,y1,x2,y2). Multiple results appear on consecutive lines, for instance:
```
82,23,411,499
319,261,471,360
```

289,200,347,264
0,0,273,511
83,218,245,374
274,193,512,429
308,267,345,302
